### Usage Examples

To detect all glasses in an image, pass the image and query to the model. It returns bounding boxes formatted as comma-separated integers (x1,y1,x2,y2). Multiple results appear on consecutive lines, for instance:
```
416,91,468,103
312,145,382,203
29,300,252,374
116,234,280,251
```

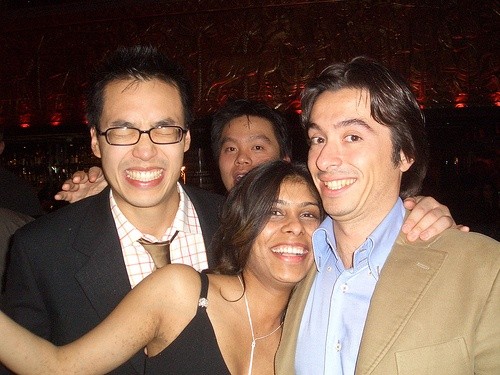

95,122,186,146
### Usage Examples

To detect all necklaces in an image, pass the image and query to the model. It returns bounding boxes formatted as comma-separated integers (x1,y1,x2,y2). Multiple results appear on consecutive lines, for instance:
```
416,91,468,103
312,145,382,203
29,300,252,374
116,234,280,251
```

238,275,284,375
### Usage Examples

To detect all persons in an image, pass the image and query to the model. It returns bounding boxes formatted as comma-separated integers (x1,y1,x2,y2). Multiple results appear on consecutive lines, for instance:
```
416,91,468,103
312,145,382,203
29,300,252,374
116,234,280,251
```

275,56,500,374
0,49,226,374
0,133,37,276
1,160,470,375
55,101,290,203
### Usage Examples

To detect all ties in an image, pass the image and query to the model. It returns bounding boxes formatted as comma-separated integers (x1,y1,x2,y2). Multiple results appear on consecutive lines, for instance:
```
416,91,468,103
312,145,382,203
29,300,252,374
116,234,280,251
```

137,231,178,270
